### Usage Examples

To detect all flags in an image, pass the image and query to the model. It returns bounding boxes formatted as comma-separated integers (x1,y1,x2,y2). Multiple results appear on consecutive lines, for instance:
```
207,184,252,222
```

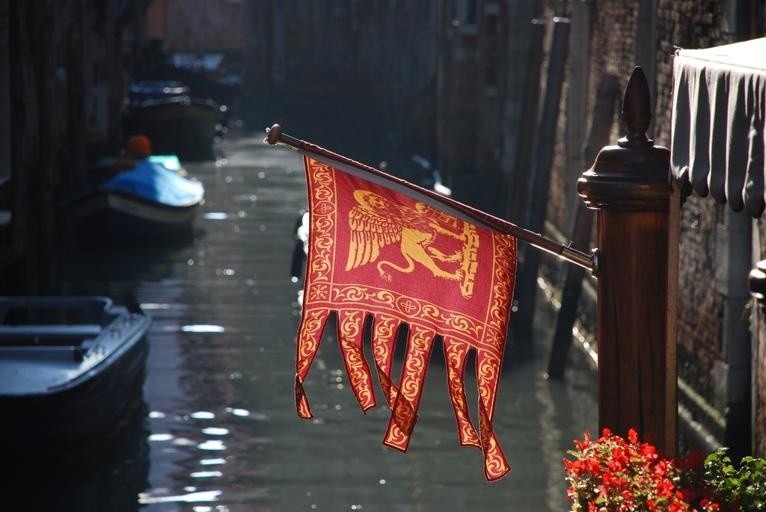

291,139,520,481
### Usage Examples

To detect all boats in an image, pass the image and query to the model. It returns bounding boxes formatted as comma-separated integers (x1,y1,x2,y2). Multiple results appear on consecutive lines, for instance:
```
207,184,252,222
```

119,48,235,142
1,283,151,422
53,155,209,245
